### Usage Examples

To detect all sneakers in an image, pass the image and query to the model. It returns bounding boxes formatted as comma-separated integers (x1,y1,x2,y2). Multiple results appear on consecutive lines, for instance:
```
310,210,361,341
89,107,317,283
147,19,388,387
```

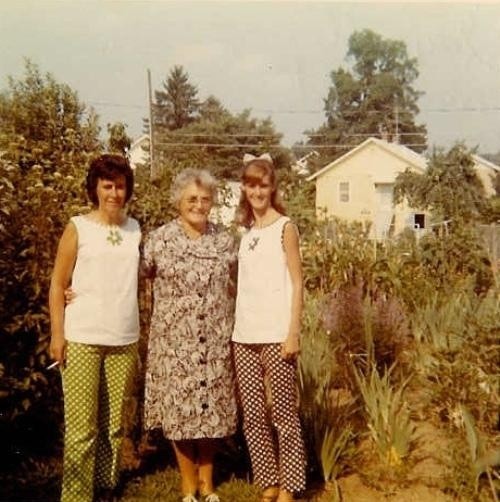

179,493,222,502
261,486,297,502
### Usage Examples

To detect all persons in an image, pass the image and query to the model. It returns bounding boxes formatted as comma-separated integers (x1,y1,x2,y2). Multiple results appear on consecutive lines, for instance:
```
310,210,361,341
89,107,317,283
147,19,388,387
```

49,153,150,502
227,156,309,502
61,165,254,500
